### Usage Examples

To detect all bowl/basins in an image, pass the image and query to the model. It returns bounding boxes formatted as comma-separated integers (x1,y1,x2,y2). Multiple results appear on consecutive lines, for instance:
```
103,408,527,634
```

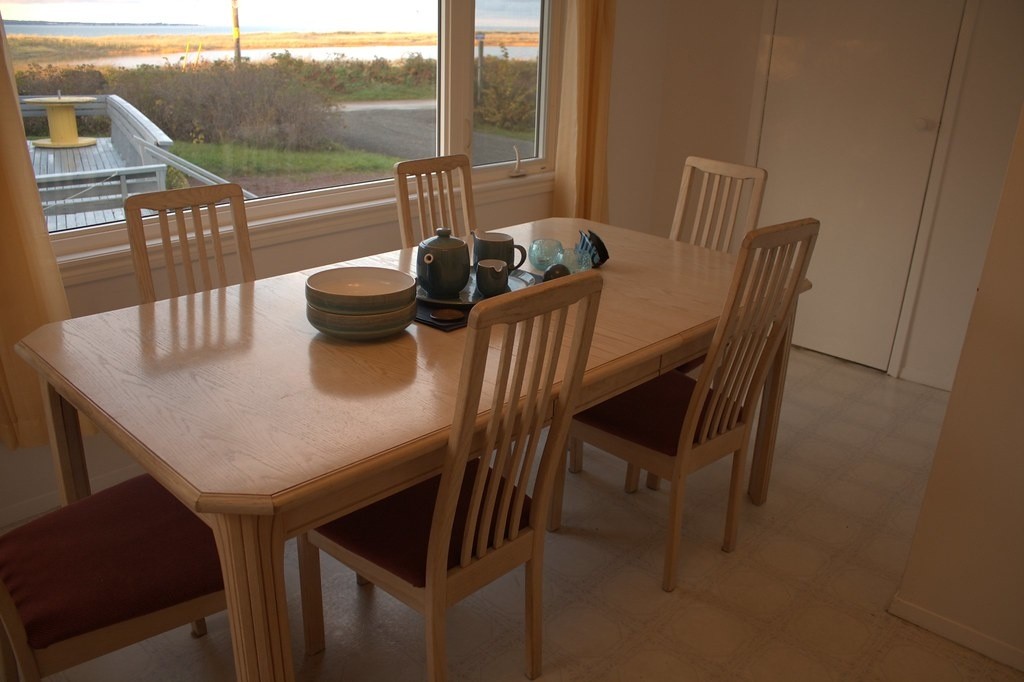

306,266,417,340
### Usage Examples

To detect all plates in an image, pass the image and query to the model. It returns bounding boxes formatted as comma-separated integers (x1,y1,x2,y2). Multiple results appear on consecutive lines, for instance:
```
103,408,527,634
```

430,310,465,321
415,266,535,308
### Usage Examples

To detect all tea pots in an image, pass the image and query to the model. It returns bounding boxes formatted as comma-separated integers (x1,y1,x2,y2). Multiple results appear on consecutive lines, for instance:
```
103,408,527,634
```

417,228,470,297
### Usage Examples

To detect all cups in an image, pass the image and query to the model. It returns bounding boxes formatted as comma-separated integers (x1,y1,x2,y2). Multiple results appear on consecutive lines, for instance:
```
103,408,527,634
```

479,260,509,298
528,238,563,270
556,248,592,275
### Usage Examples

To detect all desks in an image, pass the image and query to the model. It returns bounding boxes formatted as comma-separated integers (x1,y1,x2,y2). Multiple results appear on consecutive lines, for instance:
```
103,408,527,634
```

15,216,814,681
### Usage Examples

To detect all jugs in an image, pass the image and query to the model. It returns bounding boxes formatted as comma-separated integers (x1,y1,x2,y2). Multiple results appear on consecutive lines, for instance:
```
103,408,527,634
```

471,229,526,277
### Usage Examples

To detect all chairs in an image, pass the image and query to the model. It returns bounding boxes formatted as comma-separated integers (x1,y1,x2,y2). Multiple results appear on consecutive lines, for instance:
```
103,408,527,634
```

394,153,478,251
649,156,767,489
297,268,610,682
125,183,256,303
543,215,821,592
